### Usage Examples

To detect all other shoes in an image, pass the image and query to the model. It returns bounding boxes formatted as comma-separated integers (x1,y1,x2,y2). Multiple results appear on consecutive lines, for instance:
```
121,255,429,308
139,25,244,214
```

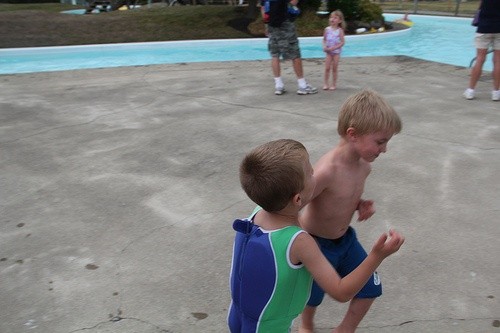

464,88,476,99
491,90,500,100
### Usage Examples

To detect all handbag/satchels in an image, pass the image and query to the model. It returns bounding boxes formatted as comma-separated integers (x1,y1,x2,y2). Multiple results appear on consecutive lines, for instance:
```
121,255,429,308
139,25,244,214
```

472,9,481,26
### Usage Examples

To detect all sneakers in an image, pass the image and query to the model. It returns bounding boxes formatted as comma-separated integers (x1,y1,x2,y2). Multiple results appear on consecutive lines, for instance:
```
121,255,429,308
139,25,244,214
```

296,84,318,94
275,85,287,94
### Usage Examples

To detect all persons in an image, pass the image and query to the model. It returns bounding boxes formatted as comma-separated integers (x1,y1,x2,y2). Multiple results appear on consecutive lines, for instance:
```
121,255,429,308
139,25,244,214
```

284,90,402,333
321,9,346,90
260,0,318,94
227,139,404,333
462,0,500,102
